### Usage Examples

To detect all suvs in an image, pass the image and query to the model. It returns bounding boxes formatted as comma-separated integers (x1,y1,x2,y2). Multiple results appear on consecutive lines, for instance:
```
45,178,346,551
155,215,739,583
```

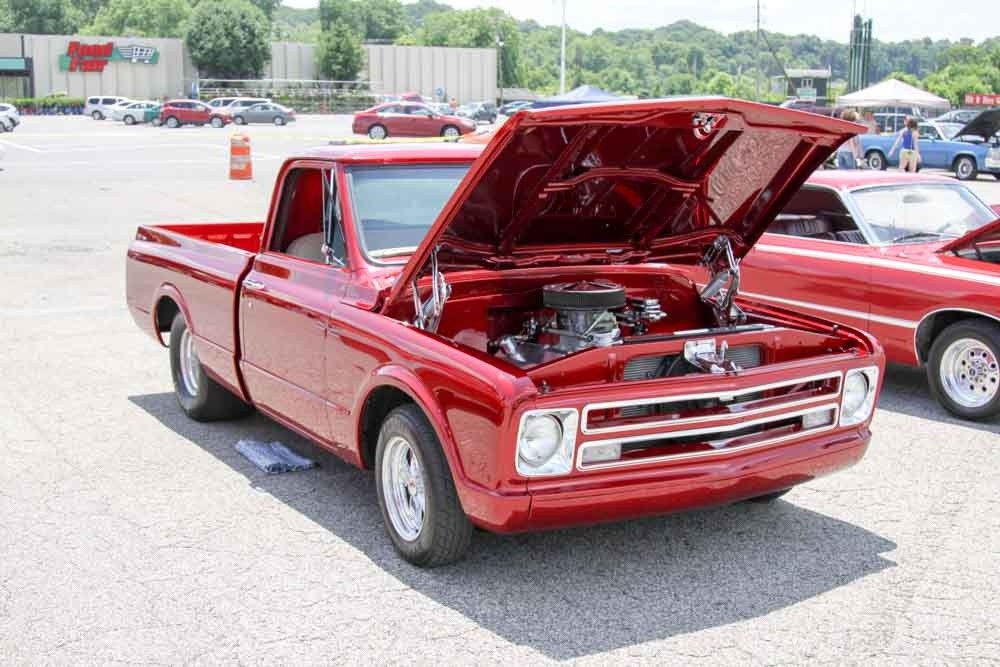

204,96,272,116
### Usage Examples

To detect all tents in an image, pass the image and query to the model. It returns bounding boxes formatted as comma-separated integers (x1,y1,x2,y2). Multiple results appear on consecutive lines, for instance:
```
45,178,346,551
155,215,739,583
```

835,79,949,132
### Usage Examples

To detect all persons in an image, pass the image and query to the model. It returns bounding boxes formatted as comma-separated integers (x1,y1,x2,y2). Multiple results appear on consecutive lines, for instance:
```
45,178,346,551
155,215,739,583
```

450,97,456,108
844,110,856,122
889,115,923,173
863,111,880,135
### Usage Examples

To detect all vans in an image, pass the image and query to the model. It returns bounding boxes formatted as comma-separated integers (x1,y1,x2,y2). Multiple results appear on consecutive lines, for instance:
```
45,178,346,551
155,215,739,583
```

85,95,129,121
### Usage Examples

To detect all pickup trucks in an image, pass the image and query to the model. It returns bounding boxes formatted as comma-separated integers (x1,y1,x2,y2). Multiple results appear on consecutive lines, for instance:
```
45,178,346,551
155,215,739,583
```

123,96,889,571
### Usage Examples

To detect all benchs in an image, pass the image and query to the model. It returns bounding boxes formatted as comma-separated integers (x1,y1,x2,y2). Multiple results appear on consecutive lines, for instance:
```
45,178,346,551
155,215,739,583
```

287,226,430,262
764,217,832,236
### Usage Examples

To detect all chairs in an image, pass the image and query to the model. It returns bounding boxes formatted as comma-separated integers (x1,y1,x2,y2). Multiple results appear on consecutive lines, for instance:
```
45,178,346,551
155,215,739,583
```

802,233,836,240
837,228,892,245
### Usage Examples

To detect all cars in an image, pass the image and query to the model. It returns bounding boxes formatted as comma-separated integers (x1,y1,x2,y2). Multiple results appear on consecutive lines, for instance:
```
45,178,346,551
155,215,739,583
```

729,169,1000,424
110,98,164,127
778,97,1000,182
351,100,478,143
158,99,234,130
0,103,22,134
381,90,537,126
231,102,297,127
